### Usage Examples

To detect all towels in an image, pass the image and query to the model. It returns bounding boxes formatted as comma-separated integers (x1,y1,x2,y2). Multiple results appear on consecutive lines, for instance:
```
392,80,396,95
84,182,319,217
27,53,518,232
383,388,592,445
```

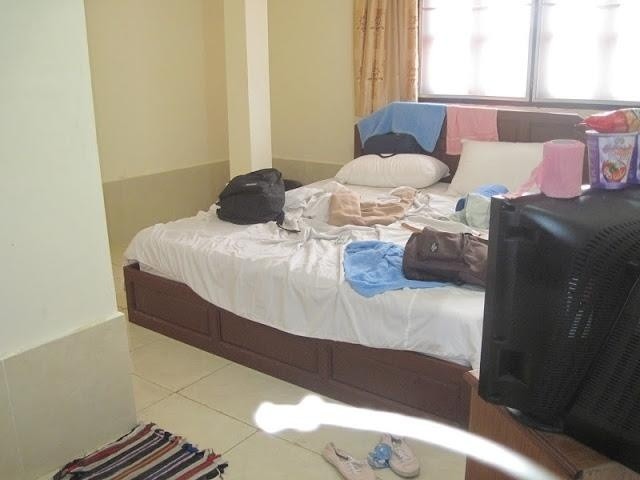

357,102,447,153
446,105,499,155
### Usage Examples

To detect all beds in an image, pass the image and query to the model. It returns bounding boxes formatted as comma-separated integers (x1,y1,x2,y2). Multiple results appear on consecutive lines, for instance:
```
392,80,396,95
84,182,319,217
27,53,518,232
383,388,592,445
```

122,102,589,432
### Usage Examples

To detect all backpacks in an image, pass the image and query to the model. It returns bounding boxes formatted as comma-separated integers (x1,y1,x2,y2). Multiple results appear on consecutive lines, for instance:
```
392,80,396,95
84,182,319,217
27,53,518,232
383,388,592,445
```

403,227,489,288
216,167,300,233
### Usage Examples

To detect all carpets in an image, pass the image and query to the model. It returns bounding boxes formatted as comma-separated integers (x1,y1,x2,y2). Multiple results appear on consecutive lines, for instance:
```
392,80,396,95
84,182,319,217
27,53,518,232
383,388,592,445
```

53,423,228,480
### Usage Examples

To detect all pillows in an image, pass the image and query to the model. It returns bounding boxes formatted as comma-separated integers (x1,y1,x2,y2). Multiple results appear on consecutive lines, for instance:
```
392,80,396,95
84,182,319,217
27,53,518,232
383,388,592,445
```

444,138,544,197
333,153,451,189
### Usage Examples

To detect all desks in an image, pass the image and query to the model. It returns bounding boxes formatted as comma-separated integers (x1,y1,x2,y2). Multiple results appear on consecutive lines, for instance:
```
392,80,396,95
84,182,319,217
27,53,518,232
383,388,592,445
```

462,369,639,479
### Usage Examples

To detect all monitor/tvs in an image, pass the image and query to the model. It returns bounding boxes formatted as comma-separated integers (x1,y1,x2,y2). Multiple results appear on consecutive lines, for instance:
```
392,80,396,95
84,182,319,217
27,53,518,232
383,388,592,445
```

476,183,640,477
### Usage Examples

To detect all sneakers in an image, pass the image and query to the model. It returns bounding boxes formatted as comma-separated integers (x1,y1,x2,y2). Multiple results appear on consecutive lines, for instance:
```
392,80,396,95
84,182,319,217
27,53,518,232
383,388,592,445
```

321,442,377,480
381,433,420,478
